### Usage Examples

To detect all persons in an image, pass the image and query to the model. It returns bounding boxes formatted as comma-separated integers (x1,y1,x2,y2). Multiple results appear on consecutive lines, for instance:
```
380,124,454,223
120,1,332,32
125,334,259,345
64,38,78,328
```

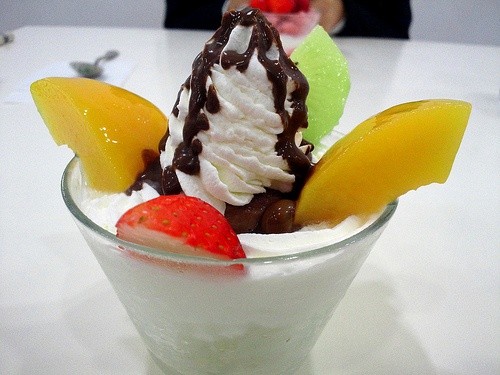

162,0,413,41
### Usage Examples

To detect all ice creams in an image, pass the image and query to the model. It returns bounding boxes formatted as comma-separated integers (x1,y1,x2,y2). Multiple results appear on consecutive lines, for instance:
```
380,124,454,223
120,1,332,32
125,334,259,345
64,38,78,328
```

28,4,473,375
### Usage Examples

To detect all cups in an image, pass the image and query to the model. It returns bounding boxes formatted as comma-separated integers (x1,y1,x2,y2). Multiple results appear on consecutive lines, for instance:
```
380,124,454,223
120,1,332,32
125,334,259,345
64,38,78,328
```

60,128,399,375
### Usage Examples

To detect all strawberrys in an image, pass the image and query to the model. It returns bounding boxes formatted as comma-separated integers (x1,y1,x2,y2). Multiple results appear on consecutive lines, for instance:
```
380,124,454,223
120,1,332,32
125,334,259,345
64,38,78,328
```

114,195,248,272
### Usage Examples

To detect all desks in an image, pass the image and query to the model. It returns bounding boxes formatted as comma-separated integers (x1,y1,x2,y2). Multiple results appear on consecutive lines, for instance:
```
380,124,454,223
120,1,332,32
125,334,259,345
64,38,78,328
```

1,25,499,375
